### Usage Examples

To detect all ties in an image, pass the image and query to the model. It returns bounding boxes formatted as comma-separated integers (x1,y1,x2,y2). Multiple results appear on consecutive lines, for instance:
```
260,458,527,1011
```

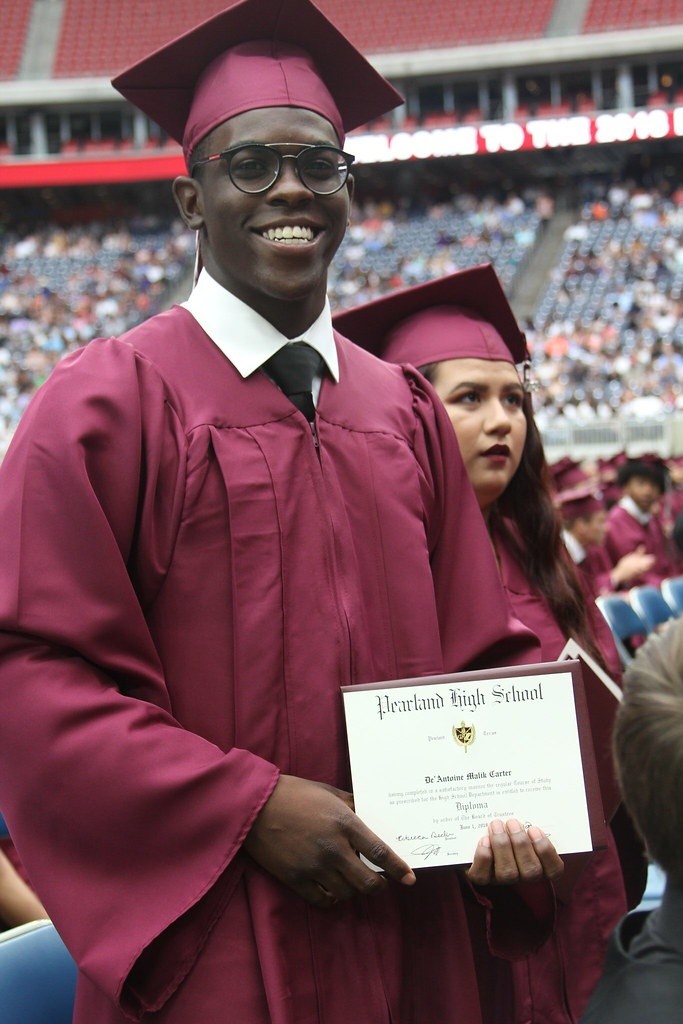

263,343,326,422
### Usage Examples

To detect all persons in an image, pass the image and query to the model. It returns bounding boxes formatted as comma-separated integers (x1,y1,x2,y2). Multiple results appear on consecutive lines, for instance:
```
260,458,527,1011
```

576,615,683,1023
0,35,629,1024
0,163,683,930
379,301,623,1024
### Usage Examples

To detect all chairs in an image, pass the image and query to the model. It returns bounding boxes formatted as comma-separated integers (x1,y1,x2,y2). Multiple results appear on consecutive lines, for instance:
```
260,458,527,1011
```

594,576,683,669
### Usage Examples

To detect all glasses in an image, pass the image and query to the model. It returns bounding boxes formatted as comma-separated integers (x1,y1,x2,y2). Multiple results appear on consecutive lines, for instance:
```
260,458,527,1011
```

191,142,355,195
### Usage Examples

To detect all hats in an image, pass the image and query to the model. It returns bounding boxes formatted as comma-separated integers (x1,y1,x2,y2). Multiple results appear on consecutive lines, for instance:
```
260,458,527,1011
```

331,263,532,394
111,0,406,173
546,449,683,522
